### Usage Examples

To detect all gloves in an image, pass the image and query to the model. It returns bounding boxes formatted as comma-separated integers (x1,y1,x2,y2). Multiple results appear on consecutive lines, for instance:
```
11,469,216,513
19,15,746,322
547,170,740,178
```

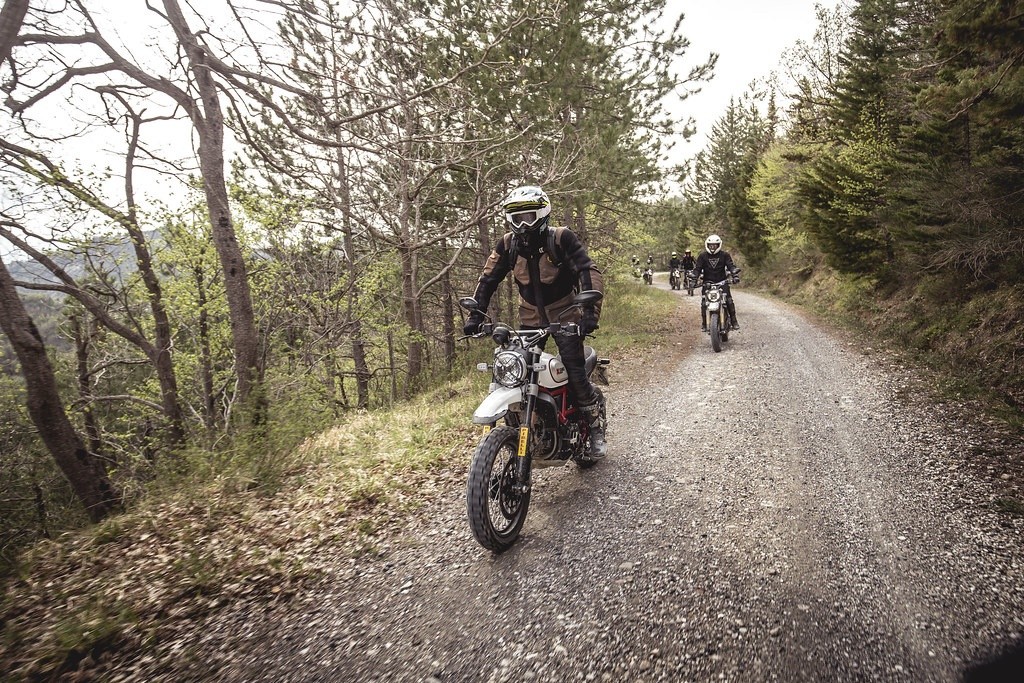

578,319,597,336
730,266,740,284
690,269,700,288
463,322,482,340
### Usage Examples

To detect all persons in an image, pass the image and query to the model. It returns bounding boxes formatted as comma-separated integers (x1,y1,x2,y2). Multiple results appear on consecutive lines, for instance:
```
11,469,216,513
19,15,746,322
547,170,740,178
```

461,186,608,459
667,250,682,284
679,249,698,288
642,259,651,277
690,234,740,331
631,255,639,272
647,256,653,269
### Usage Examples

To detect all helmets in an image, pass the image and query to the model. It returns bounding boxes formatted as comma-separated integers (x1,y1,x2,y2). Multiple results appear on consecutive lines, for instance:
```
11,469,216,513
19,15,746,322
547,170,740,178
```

685,249,691,254
672,252,676,255
705,235,723,255
502,186,551,247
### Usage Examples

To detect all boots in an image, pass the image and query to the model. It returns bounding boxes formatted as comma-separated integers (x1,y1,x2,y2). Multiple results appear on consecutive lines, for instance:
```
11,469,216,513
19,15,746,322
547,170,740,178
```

728,303,740,330
700,306,707,332
577,392,608,457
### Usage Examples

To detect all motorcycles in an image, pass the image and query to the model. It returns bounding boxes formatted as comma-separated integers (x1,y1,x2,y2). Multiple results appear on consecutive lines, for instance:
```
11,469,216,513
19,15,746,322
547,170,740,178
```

455,289,610,554
632,262,654,285
680,261,698,296
693,267,742,352
666,263,683,290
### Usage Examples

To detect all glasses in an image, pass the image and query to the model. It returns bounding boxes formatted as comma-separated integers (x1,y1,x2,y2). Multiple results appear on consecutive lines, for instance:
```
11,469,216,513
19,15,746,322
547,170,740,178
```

506,209,542,229
